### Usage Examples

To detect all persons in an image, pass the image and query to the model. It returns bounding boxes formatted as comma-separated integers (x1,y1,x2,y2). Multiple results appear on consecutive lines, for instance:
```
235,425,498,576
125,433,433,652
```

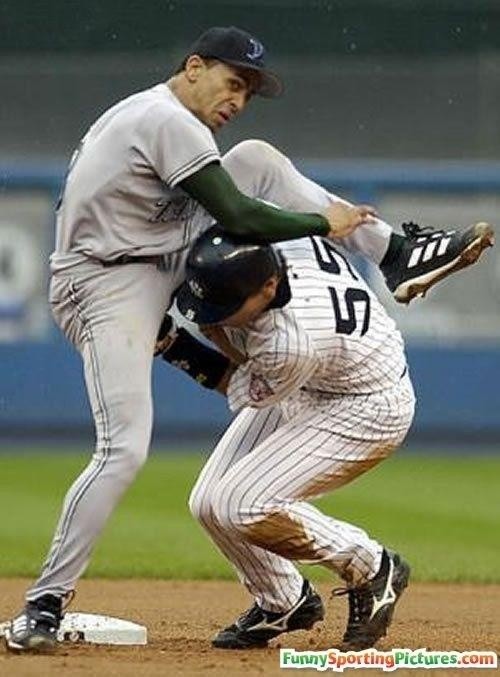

5,24,495,655
151,222,418,653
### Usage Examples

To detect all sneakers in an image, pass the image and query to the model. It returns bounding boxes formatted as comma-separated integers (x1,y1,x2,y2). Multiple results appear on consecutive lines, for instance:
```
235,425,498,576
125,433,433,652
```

384,221,495,305
9,594,65,652
334,547,409,652
211,577,326,649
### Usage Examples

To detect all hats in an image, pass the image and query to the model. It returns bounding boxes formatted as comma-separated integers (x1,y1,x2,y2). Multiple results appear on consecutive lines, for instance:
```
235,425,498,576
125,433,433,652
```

176,223,278,324
190,26,284,98
